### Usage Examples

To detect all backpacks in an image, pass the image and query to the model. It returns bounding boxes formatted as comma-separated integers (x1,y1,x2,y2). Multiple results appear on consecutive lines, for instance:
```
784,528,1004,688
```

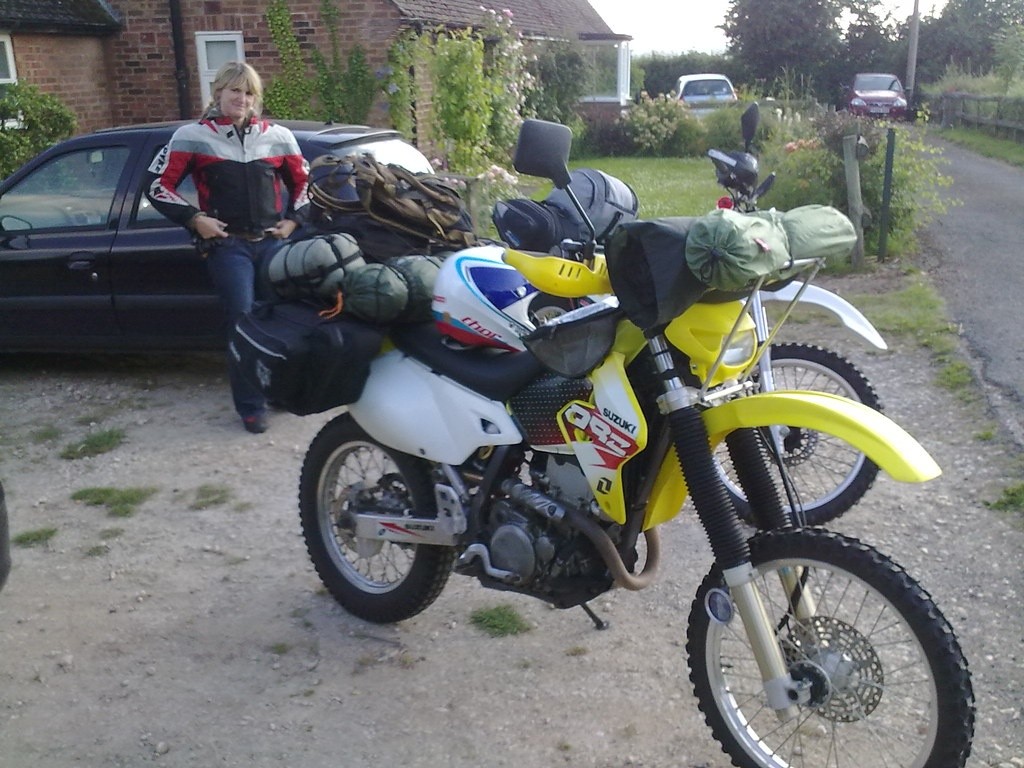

492,195,578,252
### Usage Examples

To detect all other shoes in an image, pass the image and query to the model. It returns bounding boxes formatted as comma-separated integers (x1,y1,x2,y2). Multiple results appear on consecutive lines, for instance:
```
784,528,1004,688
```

241,402,271,428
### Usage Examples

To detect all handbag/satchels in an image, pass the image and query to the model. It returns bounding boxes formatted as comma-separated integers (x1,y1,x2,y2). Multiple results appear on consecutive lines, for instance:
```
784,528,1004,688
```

306,153,479,252
228,301,380,417
546,169,640,240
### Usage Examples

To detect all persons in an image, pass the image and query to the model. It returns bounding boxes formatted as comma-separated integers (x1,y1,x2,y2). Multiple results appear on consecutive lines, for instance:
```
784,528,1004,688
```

143,63,313,433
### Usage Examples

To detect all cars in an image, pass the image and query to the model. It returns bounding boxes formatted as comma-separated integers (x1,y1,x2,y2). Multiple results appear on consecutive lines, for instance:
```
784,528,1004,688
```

667,74,738,120
0,120,437,357
844,72,907,119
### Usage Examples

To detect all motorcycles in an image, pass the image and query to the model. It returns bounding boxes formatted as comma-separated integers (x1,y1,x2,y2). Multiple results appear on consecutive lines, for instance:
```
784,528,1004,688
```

298,120,977,768
531,102,887,531
